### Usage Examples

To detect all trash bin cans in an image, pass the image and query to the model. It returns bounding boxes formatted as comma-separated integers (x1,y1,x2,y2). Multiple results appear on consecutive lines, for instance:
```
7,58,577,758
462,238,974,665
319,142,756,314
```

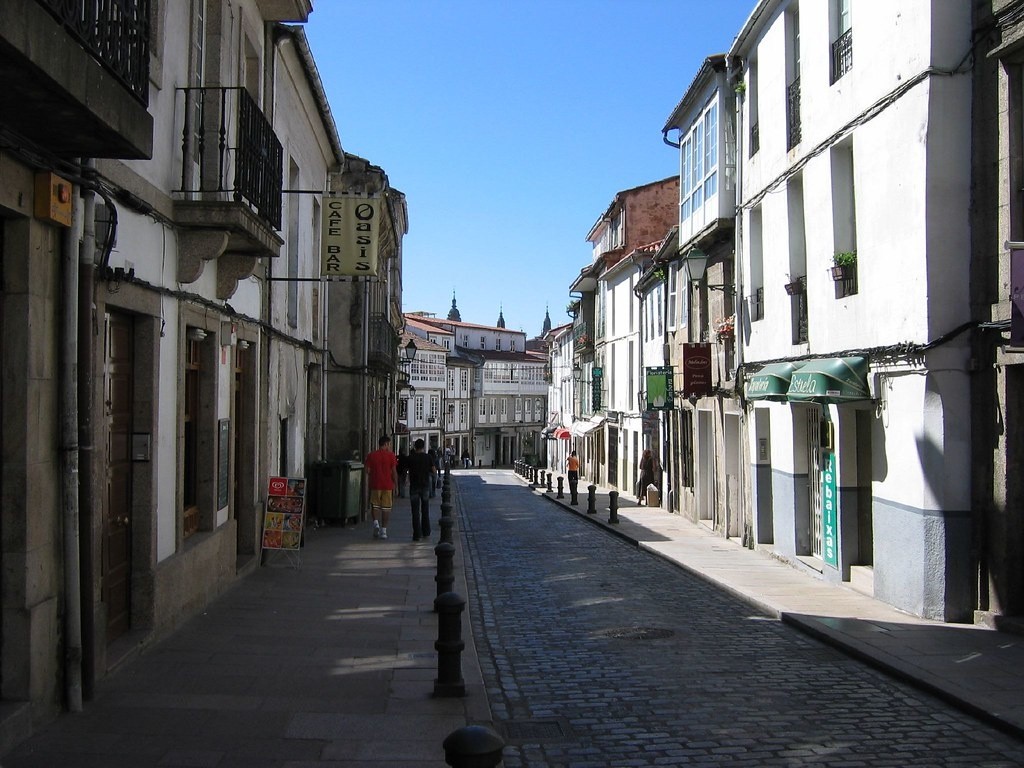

450,455,455,469
523,453,539,469
646,490,660,507
313,459,365,527
434,454,443,469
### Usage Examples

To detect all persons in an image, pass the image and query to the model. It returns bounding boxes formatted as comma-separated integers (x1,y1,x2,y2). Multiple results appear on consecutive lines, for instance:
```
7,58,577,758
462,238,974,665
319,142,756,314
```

399,439,438,542
635,449,657,506
567,452,579,494
395,445,470,499
365,436,398,539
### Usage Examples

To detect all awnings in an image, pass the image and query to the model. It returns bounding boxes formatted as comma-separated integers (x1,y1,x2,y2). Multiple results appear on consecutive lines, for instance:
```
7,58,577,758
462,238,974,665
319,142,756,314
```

748,356,871,407
541,420,604,440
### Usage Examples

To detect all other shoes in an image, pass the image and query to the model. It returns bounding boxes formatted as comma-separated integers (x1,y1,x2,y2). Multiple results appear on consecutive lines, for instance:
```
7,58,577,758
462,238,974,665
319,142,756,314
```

373,524,380,537
381,531,388,539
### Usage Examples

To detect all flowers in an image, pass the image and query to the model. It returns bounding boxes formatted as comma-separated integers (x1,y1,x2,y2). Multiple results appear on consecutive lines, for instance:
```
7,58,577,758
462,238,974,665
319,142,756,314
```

578,335,593,344
714,315,735,345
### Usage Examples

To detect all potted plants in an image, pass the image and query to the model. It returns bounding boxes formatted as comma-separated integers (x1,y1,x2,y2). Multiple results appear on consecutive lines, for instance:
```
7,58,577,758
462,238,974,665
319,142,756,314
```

830,248,856,281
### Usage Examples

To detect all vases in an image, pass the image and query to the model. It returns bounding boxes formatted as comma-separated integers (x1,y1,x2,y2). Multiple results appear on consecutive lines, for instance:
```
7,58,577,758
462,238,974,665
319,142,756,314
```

722,330,733,340
584,341,592,345
785,281,803,296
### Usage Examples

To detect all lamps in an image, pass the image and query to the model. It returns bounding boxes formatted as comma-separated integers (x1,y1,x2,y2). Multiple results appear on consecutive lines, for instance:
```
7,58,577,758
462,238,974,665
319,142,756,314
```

572,363,593,386
513,419,524,423
681,242,736,297
400,386,416,399
398,339,418,364
189,329,207,342
237,340,250,351
